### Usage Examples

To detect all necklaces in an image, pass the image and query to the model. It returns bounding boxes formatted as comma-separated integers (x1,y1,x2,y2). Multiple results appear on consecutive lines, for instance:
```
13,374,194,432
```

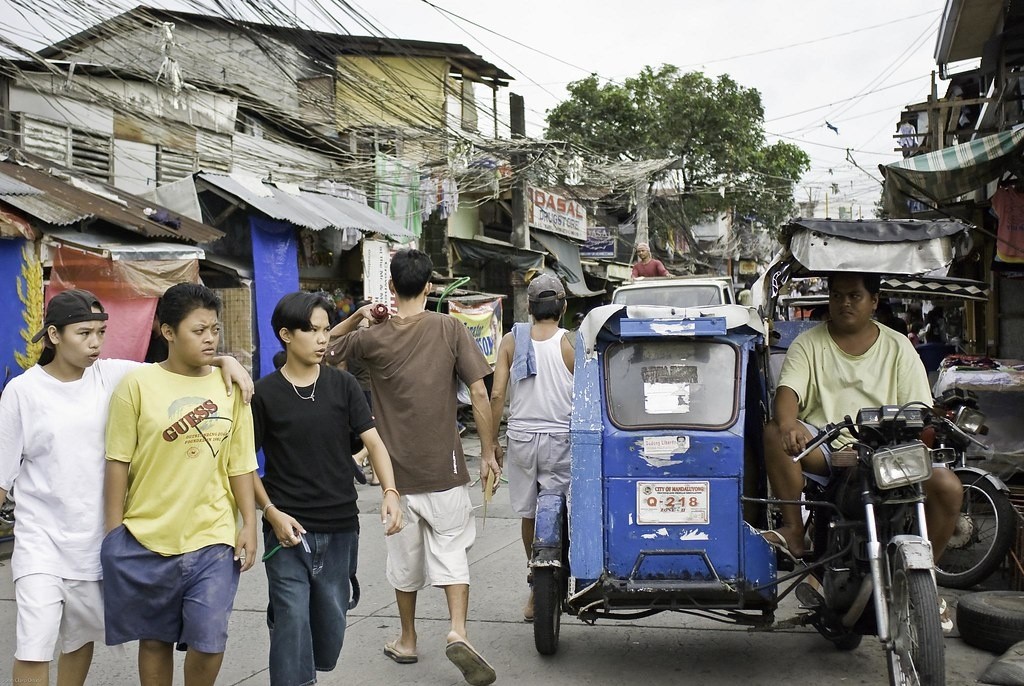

283,363,319,403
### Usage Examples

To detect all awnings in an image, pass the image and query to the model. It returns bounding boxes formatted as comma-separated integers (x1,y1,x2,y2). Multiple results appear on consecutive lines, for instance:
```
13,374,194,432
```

882,127,1024,220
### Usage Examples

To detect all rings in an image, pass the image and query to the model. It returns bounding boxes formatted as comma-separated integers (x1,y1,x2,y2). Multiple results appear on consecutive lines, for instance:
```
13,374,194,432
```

290,535,294,540
286,541,290,544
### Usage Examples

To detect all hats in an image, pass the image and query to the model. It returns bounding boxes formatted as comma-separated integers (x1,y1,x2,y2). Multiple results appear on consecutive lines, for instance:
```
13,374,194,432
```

31,288,110,343
527,274,565,301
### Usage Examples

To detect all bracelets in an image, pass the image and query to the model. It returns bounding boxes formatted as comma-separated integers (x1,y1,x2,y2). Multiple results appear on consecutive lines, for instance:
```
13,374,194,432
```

384,488,400,502
263,504,275,518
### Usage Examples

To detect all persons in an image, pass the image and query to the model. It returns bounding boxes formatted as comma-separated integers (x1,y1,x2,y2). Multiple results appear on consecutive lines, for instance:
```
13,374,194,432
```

99,281,257,686
744,272,963,637
631,243,670,278
0,290,256,686
251,294,403,686
326,248,498,686
488,269,578,623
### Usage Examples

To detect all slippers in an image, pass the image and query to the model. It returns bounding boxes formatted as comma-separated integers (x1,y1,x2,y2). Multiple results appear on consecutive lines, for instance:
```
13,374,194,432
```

444,639,496,686
384,639,417,664
937,595,953,632
759,529,799,565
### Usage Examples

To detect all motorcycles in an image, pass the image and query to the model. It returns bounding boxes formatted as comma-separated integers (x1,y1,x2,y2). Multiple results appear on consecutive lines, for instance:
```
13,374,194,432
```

921,385,1016,590
527,216,990,686
